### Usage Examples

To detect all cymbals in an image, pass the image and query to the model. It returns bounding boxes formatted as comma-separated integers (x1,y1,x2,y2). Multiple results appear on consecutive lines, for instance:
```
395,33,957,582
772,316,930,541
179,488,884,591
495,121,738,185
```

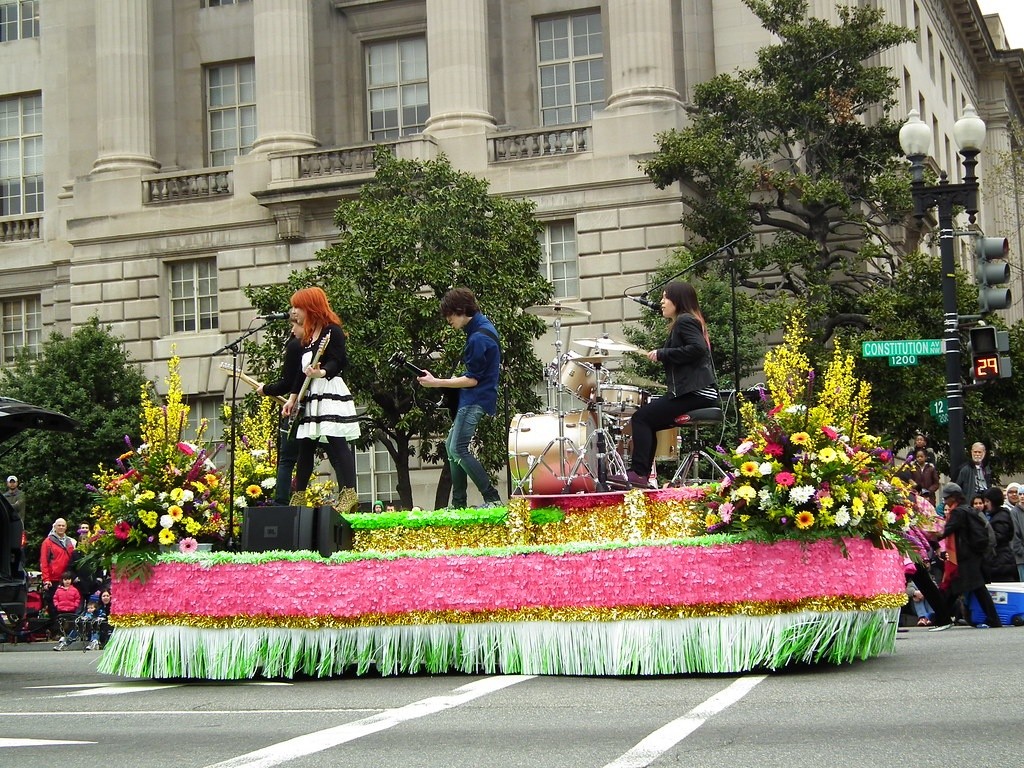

523,305,591,318
571,337,640,352
568,355,627,362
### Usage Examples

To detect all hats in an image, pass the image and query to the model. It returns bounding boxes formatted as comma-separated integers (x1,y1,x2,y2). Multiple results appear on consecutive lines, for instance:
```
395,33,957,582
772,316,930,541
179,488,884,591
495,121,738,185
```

939,482,961,497
919,488,929,496
7,476,18,482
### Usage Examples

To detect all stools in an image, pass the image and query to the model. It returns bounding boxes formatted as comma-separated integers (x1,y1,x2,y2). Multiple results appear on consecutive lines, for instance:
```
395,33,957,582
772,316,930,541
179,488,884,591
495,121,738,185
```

669,407,723,488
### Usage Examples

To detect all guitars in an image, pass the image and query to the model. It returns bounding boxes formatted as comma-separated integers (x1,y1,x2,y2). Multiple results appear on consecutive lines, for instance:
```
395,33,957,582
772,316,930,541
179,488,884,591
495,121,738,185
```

279,331,332,454
387,350,460,421
217,362,287,406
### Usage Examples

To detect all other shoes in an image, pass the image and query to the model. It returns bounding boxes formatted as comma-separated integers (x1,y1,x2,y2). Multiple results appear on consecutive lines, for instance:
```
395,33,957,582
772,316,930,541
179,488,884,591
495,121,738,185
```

444,505,468,511
59,637,64,642
474,502,504,509
976,623,991,628
928,619,954,631
927,619,931,625
917,618,927,625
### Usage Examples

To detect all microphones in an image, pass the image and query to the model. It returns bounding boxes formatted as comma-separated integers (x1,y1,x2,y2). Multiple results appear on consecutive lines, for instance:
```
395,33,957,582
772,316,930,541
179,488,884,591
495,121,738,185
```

255,312,290,319
627,294,661,311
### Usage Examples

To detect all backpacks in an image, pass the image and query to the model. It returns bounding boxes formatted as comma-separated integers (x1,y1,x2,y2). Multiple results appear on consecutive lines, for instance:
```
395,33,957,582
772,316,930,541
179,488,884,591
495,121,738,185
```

957,504,997,562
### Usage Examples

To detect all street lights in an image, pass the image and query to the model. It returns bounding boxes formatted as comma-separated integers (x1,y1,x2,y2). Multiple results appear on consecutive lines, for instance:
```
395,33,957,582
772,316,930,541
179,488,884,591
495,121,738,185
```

898,103,988,484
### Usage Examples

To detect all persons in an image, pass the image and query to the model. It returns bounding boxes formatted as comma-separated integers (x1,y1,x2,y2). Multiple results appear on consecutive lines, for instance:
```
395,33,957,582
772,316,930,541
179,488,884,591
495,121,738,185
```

41,518,113,650
373,500,423,512
897,435,1024,631
257,287,359,513
605,281,719,491
3,476,25,523
417,286,501,510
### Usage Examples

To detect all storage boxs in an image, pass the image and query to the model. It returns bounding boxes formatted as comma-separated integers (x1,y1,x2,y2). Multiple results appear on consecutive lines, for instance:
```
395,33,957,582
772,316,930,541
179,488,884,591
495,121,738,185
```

965,582,1024,626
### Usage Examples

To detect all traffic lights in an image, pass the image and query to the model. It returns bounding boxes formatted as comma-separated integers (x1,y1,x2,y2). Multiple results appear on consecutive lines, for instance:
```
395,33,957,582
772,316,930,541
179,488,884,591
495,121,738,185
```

974,236,1013,315
969,326,1003,381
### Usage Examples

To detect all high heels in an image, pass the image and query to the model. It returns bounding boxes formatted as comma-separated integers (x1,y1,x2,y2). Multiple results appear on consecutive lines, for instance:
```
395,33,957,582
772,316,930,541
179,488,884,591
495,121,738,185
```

334,486,358,513
289,491,309,506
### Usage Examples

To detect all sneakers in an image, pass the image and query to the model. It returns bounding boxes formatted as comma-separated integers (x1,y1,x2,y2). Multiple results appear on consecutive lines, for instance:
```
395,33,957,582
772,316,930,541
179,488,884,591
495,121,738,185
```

53,642,68,650
85,643,99,649
606,470,656,488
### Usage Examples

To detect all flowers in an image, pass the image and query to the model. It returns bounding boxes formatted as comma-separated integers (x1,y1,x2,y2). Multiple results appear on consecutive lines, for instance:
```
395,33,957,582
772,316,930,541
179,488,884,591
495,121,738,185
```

71,306,937,582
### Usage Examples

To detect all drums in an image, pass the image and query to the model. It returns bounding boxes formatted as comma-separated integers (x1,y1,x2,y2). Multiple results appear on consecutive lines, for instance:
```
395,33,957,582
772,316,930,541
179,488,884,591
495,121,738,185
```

600,383,643,416
550,351,610,403
507,411,600,496
613,418,681,461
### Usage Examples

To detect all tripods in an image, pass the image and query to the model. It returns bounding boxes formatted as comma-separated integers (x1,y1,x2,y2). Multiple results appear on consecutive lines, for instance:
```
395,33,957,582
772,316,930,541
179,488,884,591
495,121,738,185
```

511,309,633,495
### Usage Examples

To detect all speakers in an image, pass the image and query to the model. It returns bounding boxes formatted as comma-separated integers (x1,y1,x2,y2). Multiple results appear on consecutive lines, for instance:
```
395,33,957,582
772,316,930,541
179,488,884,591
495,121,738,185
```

241,506,355,560
